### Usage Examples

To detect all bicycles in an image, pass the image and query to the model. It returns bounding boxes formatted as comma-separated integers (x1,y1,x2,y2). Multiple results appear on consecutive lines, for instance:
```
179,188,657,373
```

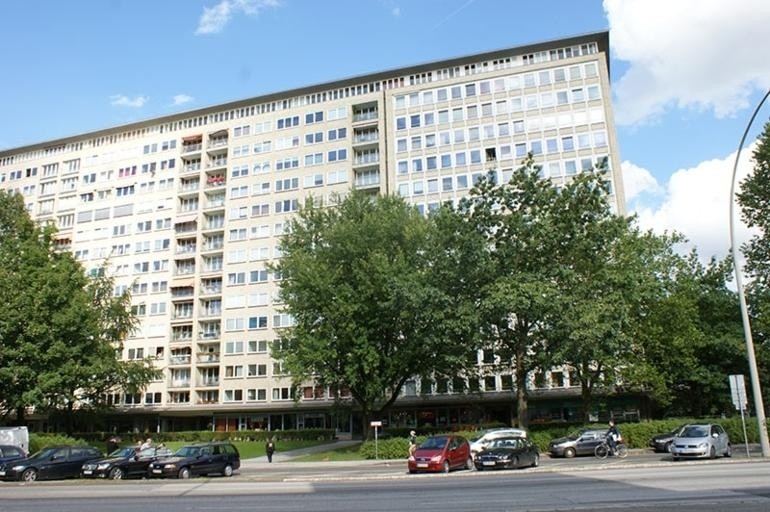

592,435,628,459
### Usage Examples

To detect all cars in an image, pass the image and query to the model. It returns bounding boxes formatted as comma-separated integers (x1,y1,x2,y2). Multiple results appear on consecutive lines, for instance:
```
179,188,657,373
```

473,436,540,470
407,434,472,476
671,423,731,459
651,422,698,454
550,427,615,457
81,444,174,481
467,428,527,457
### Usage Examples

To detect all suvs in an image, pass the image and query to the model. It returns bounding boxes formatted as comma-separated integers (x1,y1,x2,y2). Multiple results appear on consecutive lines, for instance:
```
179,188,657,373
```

149,440,241,478
0,443,26,477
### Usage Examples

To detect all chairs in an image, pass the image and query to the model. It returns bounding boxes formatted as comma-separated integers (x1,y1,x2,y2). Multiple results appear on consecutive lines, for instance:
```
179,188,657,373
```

499,442,514,448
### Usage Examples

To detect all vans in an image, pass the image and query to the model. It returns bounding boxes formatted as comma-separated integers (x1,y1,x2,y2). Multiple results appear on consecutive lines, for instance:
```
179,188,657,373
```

1,444,106,483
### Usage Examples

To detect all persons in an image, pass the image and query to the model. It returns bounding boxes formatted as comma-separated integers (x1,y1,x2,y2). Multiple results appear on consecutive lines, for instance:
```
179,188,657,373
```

408,430,417,457
136,438,165,451
604,419,623,455
265,437,275,462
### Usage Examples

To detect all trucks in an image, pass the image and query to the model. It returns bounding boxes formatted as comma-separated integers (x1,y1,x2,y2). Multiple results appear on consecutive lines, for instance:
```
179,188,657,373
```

0,426,31,457
